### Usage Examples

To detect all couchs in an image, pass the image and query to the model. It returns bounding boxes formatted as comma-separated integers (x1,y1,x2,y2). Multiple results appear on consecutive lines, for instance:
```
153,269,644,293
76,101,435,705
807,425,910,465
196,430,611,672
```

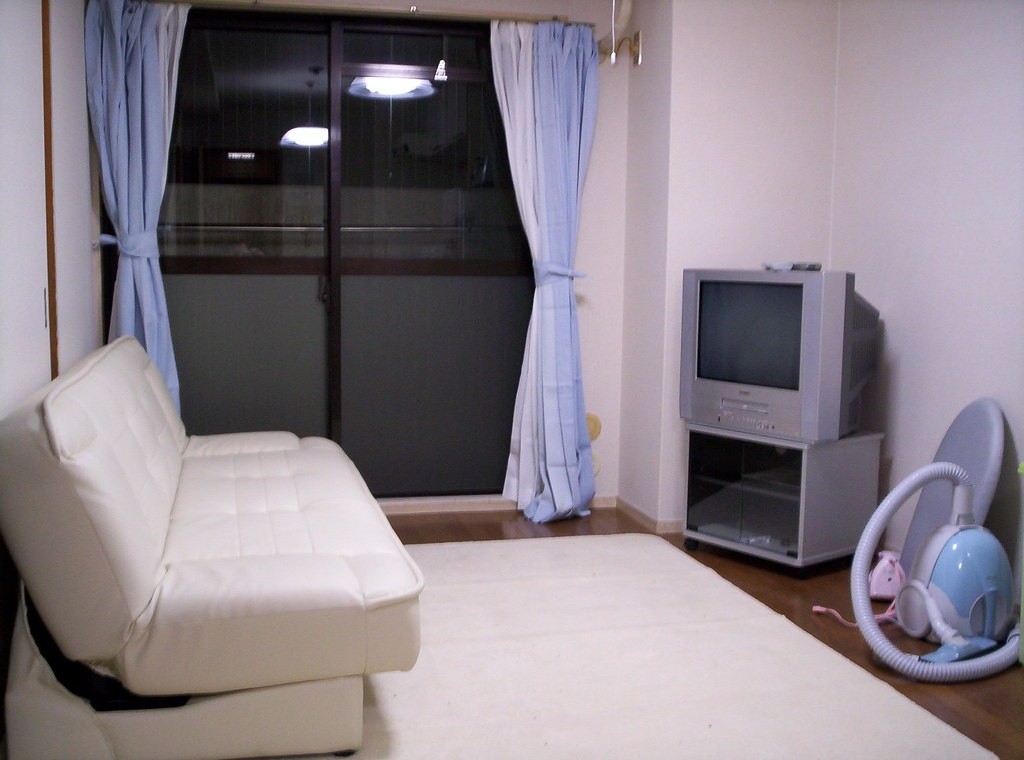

0,331,424,760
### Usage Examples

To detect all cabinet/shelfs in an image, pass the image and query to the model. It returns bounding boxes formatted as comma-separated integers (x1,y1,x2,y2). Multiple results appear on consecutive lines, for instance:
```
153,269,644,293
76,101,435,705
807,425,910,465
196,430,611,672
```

682,430,885,574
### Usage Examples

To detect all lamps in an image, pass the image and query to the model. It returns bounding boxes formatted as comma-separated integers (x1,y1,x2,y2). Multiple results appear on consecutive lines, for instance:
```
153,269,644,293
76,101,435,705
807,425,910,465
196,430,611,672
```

279,79,331,148
347,34,438,100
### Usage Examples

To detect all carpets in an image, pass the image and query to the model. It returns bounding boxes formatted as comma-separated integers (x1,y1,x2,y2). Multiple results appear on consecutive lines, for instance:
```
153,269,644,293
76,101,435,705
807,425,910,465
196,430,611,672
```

307,534,1001,760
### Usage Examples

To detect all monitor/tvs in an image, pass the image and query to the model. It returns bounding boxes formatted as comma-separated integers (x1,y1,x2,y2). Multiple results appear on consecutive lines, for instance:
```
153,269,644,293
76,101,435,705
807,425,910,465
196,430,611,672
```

681,268,879,444
